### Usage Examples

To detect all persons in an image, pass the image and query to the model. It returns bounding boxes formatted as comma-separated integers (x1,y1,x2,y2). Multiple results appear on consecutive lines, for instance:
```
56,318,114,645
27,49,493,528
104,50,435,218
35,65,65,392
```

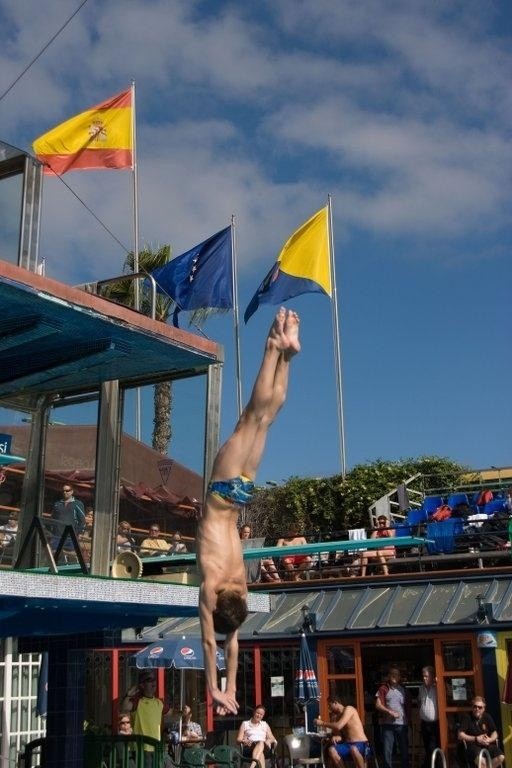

236,705,276,766
194,305,302,717
122,668,202,766
1,482,187,556
240,504,475,583
314,694,371,767
376,666,505,767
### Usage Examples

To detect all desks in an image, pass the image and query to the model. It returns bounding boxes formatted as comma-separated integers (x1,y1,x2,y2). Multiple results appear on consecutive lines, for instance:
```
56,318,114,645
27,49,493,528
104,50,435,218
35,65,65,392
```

307,736,335,768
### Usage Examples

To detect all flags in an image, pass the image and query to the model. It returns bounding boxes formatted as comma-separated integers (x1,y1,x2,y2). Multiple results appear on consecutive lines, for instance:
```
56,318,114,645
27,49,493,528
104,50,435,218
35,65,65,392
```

29,85,136,177
142,223,235,317
243,203,334,327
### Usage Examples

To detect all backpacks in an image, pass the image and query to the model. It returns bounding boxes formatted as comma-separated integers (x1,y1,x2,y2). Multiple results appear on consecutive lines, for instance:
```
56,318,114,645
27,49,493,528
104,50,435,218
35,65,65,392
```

476,489,493,505
428,502,475,523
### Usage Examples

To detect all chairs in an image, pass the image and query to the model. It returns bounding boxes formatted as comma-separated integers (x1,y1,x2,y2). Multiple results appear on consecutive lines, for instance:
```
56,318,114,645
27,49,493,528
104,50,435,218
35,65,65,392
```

1,491,511,580
321,738,379,768
171,730,207,767
211,745,261,767
284,734,320,767
181,747,216,767
462,738,503,768
240,742,278,768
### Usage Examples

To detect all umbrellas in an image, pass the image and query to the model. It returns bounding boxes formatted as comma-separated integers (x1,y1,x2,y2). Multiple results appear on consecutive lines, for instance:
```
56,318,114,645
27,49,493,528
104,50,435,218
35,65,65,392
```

291,631,323,738
127,632,237,734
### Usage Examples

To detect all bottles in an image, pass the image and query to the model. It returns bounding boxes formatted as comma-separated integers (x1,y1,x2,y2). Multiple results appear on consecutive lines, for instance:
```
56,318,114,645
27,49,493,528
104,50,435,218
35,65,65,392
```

317,715,323,732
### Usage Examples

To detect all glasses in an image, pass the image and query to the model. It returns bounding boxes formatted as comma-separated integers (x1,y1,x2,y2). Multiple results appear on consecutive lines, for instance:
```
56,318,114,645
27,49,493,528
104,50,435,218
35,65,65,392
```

473,705,483,709
